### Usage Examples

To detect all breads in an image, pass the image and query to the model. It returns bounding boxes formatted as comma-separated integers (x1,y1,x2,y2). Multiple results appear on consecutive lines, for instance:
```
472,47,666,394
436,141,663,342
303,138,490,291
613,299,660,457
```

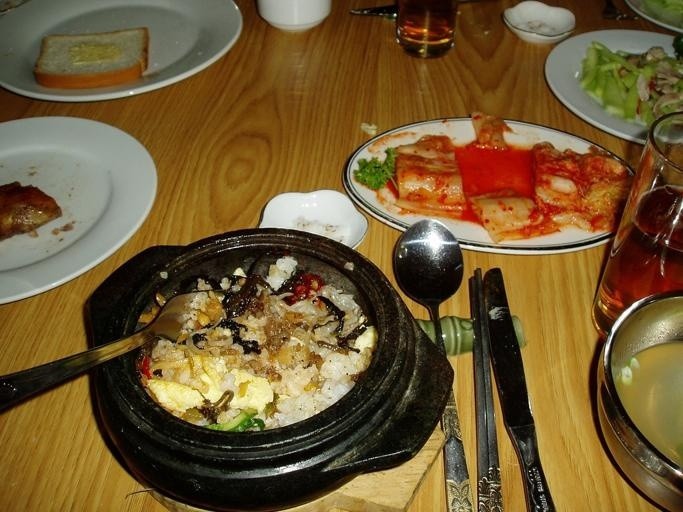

33,29,149,88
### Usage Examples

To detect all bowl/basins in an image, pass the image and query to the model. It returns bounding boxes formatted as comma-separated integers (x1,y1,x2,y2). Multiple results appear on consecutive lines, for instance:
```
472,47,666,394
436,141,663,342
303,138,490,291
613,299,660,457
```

504,1,577,43
83,230,454,512
594,288,682,512
254,189,369,252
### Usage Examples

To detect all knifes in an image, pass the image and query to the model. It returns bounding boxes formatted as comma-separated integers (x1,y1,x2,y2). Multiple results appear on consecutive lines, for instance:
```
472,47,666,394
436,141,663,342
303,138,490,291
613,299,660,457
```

481,268,558,512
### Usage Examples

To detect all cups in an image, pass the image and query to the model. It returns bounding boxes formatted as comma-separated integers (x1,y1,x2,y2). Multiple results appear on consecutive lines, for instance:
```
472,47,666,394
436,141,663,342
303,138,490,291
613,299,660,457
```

257,0,332,35
589,112,683,341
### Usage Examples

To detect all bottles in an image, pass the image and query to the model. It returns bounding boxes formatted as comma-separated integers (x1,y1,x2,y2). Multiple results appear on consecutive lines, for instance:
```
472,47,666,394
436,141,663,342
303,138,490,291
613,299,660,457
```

394,1,459,59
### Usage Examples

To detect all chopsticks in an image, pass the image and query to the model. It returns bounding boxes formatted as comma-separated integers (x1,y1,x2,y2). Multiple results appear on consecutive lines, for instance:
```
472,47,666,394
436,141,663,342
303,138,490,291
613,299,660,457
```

468,268,503,512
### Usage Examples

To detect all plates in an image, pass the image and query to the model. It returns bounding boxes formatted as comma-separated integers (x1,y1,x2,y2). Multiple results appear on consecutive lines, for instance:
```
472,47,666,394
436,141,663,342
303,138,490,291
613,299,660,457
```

0,115,157,306
0,0,244,104
624,1,683,34
339,117,634,256
542,28,683,145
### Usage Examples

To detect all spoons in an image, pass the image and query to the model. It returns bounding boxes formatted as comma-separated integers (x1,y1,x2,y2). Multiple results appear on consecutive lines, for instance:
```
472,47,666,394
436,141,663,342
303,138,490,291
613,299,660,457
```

0,288,230,415
392,220,481,511
